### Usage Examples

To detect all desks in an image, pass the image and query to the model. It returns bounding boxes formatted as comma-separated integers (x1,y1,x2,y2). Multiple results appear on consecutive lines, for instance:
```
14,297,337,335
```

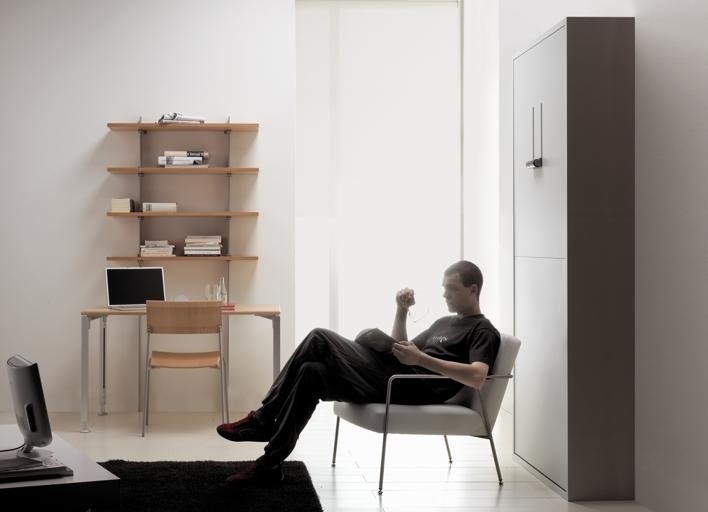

79,301,281,433
0,424,119,512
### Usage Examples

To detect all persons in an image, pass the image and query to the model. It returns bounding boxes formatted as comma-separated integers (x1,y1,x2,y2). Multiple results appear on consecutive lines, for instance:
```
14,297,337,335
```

216,259,501,488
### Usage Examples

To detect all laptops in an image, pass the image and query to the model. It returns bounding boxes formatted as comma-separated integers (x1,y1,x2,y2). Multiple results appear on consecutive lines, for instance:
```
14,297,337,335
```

105,266,166,311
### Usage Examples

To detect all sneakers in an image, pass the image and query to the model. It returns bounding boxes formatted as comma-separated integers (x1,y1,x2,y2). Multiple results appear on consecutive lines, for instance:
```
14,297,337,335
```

217,410,279,443
227,459,284,485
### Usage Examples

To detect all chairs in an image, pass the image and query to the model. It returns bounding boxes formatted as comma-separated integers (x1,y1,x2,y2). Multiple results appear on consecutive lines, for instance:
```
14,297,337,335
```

141,300,230,437
331,331,521,495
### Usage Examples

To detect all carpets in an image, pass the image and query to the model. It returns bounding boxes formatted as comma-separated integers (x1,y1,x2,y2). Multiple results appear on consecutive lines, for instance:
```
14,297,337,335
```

0,461,322,512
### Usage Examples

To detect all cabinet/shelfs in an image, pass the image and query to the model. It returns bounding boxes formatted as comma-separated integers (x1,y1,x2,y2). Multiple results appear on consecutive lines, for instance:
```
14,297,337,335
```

105,123,261,257
511,18,634,503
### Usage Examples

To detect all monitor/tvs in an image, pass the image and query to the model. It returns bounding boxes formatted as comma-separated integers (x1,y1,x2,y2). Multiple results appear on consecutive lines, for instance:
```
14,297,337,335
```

0,353,74,483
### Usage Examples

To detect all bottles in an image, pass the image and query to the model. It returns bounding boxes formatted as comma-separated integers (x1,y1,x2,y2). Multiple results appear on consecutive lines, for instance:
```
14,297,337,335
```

217,277,228,306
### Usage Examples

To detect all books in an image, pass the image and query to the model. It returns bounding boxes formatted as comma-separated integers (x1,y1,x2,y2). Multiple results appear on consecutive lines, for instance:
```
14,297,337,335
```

109,112,224,257
0,455,43,471
221,304,236,312
